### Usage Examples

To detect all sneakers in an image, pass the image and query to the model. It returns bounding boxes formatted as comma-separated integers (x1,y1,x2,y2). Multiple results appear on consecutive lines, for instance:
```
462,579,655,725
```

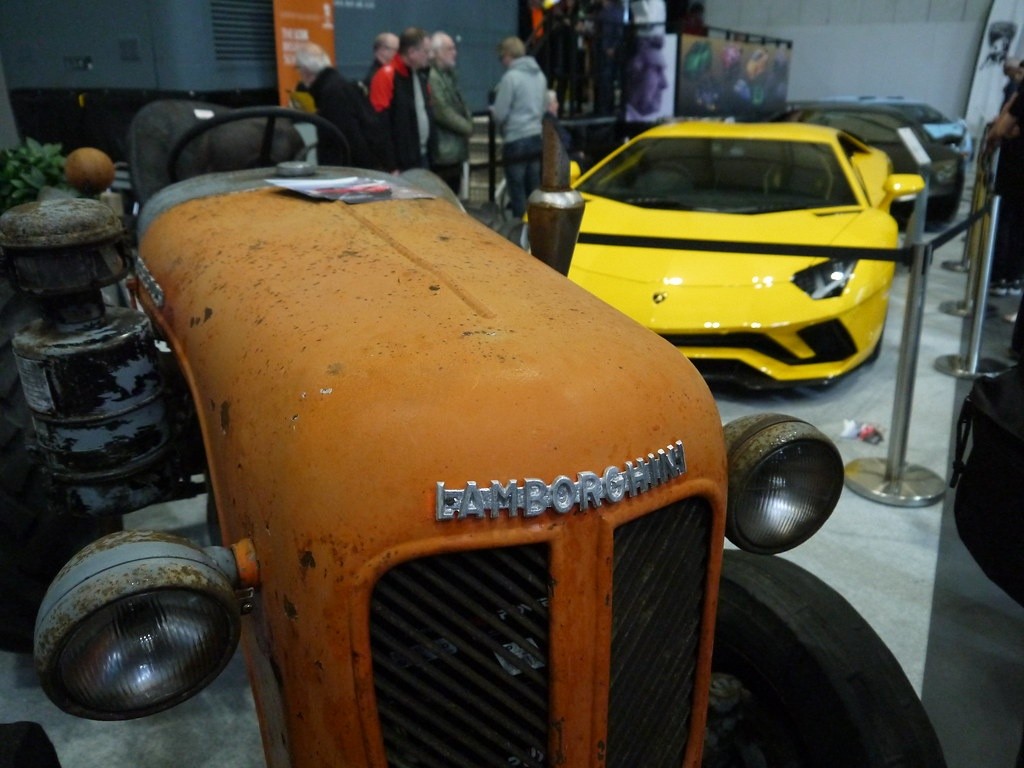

989,282,1021,296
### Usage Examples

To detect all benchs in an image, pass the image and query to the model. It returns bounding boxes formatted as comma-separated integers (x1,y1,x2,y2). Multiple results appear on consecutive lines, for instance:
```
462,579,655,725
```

634,139,834,211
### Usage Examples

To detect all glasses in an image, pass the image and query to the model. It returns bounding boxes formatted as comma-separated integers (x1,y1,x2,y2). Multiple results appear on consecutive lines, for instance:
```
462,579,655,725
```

498,54,506,61
293,65,301,70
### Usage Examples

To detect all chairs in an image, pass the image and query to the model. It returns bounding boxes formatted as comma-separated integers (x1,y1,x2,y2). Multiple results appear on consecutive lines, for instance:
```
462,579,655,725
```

126,100,297,205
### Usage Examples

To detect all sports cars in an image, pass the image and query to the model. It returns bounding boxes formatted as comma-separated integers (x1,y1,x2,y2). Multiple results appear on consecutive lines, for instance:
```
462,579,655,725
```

517,121,924,394
747,99,969,224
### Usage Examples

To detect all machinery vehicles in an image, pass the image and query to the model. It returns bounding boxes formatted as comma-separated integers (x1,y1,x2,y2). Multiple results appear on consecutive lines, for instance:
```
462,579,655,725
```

2,98,946,768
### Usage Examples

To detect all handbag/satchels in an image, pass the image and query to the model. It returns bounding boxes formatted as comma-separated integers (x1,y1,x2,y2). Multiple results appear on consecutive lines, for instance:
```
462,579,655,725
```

949,361,1024,607
431,133,469,167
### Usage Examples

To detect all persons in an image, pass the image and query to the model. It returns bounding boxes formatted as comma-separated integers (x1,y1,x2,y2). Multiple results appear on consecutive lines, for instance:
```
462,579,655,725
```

486,35,549,220
978,54,1024,286
542,89,588,172
427,30,473,196
369,26,434,178
285,42,357,165
363,31,400,87
